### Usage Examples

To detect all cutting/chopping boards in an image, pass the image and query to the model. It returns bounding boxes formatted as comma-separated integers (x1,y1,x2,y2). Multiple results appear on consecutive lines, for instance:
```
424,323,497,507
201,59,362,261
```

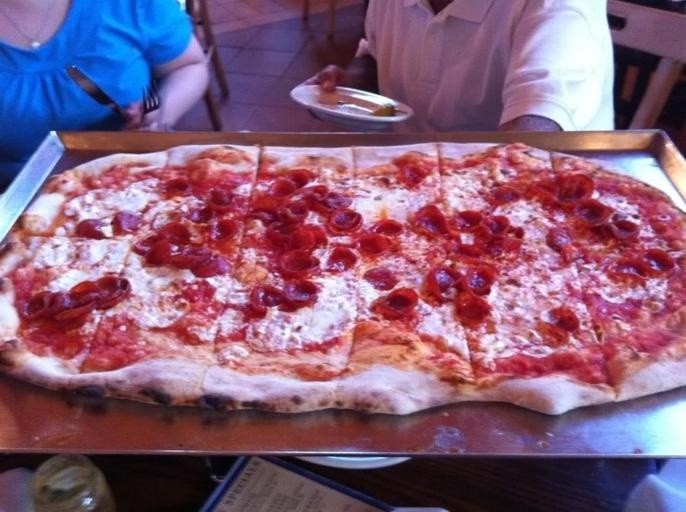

3,127,685,452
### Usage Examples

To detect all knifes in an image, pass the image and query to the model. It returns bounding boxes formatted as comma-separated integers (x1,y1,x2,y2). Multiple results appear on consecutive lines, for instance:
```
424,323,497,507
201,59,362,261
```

66,67,131,124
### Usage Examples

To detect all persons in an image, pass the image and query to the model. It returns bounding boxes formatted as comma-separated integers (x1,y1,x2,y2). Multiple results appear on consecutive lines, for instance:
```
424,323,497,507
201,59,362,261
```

1,0,211,192
301,0,619,136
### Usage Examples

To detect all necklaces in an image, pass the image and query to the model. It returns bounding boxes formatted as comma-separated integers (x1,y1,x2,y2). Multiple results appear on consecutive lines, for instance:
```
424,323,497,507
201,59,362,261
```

1,0,56,49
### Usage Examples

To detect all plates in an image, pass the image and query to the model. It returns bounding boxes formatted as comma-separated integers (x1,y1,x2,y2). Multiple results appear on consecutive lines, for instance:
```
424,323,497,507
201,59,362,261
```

290,83,414,131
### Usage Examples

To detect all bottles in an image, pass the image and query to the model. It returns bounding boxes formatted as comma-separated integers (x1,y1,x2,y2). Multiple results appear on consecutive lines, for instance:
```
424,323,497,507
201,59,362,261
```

29,452,112,511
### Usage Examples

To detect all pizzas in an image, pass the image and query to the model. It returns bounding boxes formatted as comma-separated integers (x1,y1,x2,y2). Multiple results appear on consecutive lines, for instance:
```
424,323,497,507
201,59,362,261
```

0,141,686,416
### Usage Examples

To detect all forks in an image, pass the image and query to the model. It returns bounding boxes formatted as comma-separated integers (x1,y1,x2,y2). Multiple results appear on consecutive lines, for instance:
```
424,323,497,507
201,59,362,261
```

141,80,164,123
